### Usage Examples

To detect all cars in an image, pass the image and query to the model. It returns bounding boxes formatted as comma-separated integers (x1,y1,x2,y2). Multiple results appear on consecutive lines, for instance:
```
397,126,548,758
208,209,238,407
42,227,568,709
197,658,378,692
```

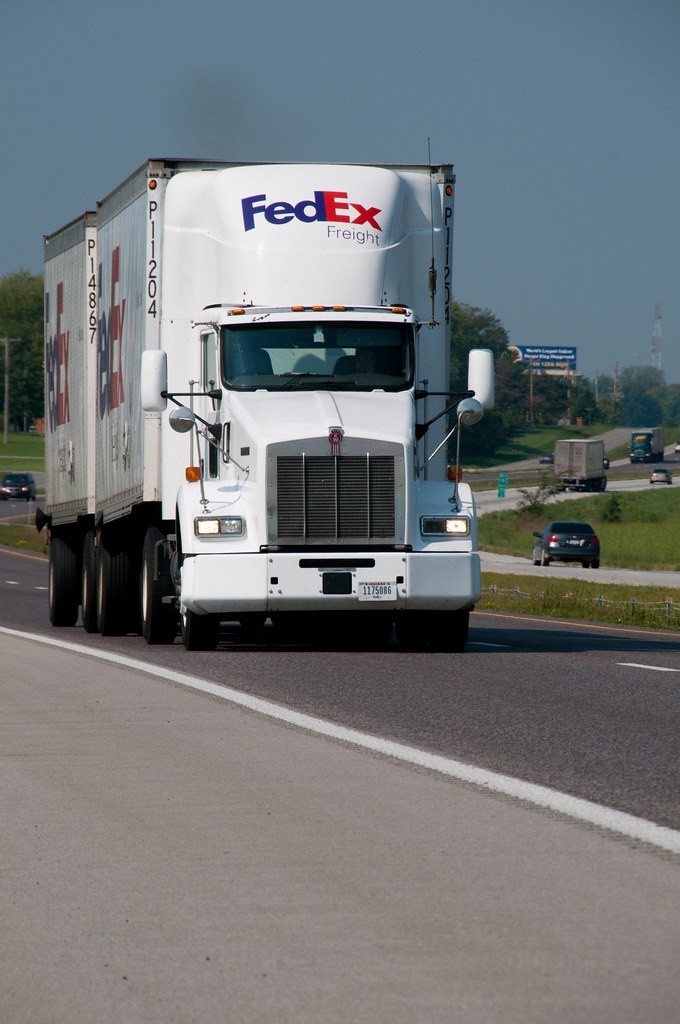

650,469,673,484
531,521,600,569
538,454,554,463
559,417,575,425
674,441,680,453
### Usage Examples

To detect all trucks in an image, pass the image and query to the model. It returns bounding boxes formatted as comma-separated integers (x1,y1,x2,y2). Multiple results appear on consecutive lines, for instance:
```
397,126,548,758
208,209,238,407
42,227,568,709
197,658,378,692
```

554,438,609,491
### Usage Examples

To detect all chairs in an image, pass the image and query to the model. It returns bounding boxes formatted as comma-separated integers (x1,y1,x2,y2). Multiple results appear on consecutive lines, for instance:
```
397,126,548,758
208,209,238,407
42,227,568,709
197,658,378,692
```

334,356,375,376
230,348,274,379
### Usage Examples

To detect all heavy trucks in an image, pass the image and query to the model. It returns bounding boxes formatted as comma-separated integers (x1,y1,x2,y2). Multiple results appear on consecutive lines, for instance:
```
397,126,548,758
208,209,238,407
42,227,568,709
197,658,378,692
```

628,427,664,463
36,158,499,651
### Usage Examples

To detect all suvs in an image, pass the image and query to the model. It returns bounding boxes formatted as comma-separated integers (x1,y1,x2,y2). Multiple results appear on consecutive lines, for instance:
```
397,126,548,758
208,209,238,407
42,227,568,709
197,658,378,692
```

0,473,35,501
